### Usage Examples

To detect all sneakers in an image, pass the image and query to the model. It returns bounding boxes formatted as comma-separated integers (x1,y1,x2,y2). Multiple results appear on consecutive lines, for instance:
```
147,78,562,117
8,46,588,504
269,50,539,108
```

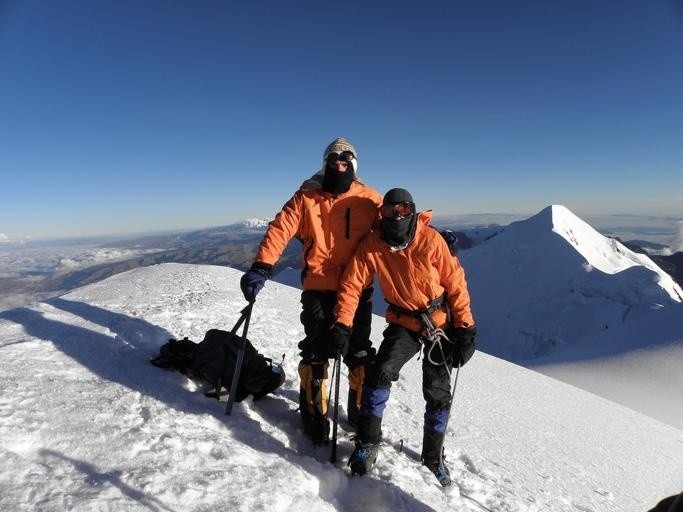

425,461,452,487
306,423,335,448
347,438,378,473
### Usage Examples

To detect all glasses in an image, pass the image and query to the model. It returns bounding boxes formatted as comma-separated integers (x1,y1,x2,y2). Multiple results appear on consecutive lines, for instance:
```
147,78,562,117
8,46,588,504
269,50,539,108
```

380,203,415,219
325,151,356,163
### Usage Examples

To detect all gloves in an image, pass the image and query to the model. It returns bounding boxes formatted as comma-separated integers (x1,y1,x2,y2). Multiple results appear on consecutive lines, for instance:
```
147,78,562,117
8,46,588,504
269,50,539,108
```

329,321,353,359
447,324,478,368
238,259,274,302
439,229,458,249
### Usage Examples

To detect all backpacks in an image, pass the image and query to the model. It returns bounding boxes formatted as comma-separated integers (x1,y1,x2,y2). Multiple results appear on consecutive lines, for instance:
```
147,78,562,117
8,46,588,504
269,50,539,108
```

151,328,286,402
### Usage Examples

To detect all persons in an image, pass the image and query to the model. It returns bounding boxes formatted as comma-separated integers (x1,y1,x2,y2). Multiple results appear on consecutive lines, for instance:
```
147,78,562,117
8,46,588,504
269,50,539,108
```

315,187,477,490
237,136,460,447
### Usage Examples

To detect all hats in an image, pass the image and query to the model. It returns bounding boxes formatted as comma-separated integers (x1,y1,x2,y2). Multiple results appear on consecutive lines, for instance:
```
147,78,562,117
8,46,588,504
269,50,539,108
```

322,134,358,175
383,188,413,206
